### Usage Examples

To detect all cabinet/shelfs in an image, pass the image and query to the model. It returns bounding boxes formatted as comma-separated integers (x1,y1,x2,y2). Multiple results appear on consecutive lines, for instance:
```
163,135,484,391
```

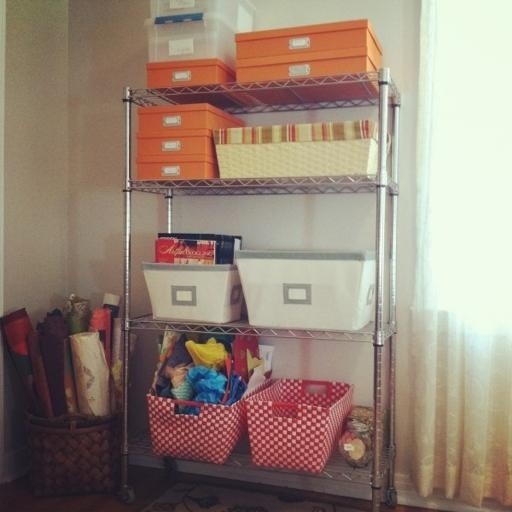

117,61,404,510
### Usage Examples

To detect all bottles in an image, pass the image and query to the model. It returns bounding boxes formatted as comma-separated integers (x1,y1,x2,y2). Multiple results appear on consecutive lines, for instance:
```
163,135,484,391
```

338,420,375,469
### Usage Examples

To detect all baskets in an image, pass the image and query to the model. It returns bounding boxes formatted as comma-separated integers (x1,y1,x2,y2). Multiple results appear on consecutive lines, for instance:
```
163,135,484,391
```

23,409,121,500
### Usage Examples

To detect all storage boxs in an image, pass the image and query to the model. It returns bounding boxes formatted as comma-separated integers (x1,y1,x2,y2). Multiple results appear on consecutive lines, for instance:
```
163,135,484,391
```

213,119,394,185
150,2,258,34
136,129,216,164
234,248,377,334
139,260,243,324
146,377,276,465
144,12,237,71
138,103,246,132
245,379,355,476
137,162,219,179
146,57,260,110
234,18,384,105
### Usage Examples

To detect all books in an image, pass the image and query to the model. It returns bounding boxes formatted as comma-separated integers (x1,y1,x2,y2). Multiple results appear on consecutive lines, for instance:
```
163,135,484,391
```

154,237,216,266
